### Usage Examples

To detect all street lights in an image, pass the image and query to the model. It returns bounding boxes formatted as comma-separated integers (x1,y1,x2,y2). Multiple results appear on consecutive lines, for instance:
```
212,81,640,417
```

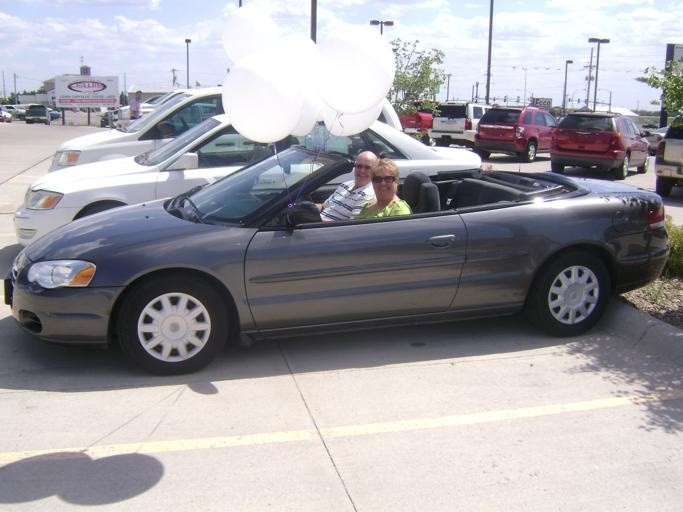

556,30,607,109
182,34,194,87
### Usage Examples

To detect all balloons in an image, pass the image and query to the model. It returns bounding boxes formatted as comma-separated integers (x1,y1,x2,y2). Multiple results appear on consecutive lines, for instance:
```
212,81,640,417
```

221,0,396,145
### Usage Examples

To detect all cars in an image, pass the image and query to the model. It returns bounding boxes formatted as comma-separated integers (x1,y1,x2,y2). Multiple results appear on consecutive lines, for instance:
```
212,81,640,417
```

12,114,482,247
118,92,186,119
112,95,162,126
0,105,12,123
49,110,60,120
646,126,669,155
642,123,658,129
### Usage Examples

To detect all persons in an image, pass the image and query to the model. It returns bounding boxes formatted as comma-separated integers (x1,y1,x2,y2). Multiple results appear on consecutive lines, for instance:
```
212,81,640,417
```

356,158,413,220
315,151,378,224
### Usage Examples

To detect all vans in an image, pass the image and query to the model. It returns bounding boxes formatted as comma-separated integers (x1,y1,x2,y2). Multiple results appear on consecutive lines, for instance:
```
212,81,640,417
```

430,101,492,148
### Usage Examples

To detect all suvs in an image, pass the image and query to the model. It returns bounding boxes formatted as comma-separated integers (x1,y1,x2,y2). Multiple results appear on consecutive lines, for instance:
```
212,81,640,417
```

100,111,112,127
551,111,650,180
48,89,404,177
25,105,50,125
5,105,26,121
655,115,683,197
475,106,558,160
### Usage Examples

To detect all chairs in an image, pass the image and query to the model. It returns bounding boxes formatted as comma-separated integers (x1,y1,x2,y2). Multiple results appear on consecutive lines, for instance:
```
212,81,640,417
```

402,171,432,209
415,183,441,213
249,145,271,165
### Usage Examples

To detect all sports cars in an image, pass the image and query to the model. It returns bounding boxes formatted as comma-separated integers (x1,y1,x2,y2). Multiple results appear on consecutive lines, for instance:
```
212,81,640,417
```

3,146,671,376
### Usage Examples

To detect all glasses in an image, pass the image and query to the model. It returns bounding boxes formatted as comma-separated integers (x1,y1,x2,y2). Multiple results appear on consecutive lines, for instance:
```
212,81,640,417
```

373,176,395,183
356,163,371,170
